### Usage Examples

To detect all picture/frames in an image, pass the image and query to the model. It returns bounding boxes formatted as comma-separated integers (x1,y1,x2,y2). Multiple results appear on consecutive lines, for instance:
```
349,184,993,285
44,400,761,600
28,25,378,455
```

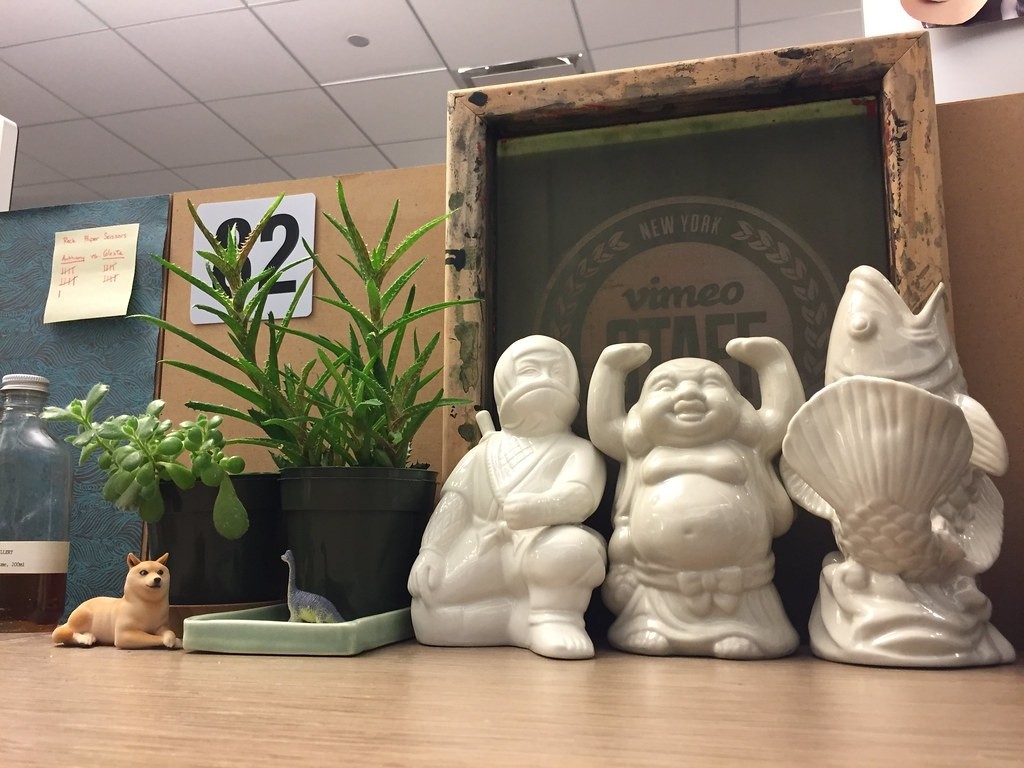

442,30,956,494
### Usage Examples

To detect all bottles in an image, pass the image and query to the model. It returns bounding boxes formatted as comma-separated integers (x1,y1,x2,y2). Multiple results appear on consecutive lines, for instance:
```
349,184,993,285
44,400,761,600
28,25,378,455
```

0,373,72,632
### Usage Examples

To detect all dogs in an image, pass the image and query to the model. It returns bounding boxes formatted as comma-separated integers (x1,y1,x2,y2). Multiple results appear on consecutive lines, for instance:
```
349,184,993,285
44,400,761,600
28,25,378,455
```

51,552,183,650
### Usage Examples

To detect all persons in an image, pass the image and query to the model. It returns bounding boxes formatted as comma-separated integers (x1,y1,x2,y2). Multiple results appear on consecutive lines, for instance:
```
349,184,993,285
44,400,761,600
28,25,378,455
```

585,337,806,661
407,333,609,658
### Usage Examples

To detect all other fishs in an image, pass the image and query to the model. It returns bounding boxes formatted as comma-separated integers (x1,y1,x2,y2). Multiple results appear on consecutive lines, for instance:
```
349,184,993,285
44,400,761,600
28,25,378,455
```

777,264,1009,580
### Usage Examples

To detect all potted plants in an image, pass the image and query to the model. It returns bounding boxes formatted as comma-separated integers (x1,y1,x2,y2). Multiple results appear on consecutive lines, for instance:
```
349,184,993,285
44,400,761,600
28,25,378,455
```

124,179,484,621
37,382,285,605
